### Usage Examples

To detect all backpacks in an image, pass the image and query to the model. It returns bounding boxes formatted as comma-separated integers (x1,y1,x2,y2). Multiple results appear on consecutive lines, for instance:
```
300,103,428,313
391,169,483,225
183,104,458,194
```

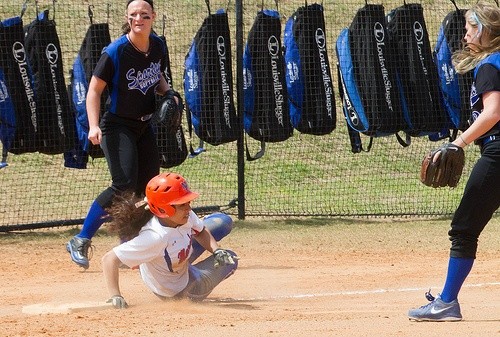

150,89,188,168
183,0,482,161
0,8,111,168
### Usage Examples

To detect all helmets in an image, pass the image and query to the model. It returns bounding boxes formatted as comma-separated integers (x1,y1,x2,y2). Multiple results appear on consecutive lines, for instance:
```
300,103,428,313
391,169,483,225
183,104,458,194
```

145,173,199,218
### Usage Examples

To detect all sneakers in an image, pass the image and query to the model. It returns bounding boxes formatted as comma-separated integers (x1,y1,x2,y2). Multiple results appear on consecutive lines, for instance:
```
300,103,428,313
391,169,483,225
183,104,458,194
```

408,290,462,321
66,234,93,269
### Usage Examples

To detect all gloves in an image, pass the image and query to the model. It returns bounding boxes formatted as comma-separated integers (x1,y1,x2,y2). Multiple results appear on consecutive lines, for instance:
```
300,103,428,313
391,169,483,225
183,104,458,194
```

106,295,128,309
212,248,241,268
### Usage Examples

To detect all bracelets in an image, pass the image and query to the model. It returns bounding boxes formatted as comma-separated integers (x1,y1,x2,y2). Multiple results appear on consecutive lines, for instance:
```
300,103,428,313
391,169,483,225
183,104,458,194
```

459,136,468,145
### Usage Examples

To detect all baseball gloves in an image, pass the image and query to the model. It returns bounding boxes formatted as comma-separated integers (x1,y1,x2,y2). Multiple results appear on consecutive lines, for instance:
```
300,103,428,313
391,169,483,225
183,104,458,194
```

156,86,182,136
420,144,466,190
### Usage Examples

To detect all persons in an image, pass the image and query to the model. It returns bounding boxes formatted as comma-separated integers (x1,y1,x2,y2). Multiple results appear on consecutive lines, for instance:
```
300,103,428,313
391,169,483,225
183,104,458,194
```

66,0,183,270
409,0,500,321
101,172,238,309
